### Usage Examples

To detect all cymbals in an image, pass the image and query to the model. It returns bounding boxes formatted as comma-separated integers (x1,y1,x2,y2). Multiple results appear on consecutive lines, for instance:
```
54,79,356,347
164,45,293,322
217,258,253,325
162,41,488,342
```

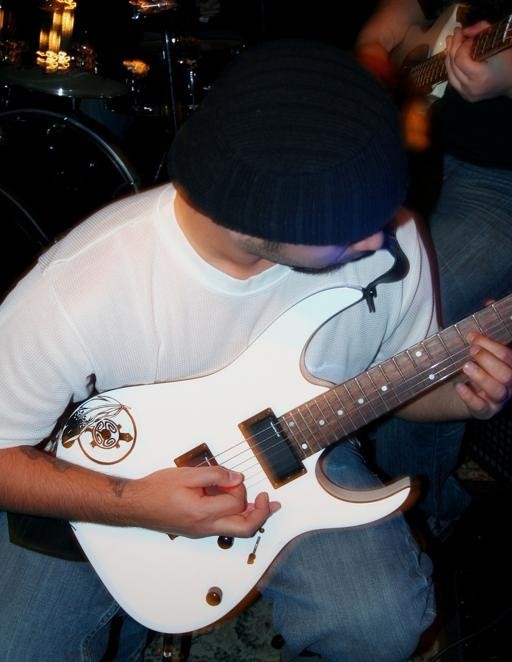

139,37,237,54
0,63,130,101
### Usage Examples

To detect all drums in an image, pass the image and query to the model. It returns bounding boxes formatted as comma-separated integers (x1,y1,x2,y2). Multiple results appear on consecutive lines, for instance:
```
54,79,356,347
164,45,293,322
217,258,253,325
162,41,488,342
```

1,96,171,254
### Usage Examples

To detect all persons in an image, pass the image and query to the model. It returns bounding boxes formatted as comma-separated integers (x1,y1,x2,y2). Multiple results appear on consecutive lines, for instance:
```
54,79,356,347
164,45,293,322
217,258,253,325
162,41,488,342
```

349,1,512,641
1,30,511,662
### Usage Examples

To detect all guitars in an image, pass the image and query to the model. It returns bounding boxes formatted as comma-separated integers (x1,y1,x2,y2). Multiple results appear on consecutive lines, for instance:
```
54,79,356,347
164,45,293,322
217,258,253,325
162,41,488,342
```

389,2,512,151
55,286,510,636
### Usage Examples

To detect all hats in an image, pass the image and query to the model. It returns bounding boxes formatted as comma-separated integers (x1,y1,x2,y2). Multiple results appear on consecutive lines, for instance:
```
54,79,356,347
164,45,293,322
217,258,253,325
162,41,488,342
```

164,32,401,239
